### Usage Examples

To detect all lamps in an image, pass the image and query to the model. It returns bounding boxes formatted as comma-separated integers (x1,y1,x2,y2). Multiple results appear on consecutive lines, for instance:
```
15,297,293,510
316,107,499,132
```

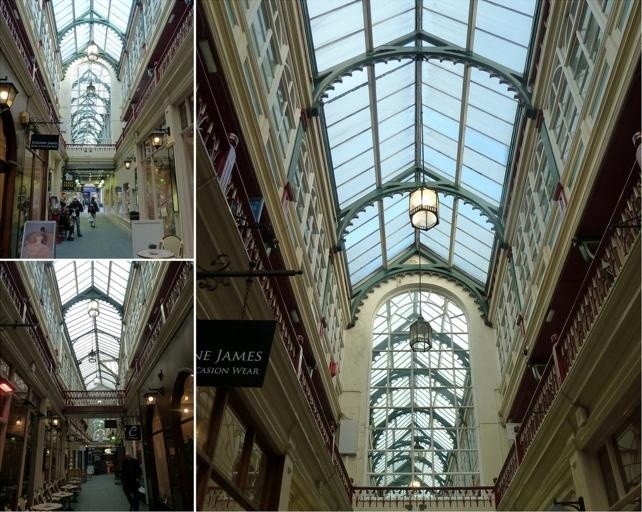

0,13,167,195
407,228,434,356
47,411,61,427
408,375,426,465
405,50,442,231
0,375,15,393
87,312,103,407
141,384,165,409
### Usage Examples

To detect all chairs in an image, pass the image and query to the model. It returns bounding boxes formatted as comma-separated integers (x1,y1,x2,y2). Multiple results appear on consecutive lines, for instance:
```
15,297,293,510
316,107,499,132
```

154,232,185,260
0,471,87,512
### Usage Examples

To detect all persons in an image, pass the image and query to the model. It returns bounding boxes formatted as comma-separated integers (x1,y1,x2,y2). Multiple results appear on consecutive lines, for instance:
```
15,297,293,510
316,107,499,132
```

120,453,142,511
50,191,101,242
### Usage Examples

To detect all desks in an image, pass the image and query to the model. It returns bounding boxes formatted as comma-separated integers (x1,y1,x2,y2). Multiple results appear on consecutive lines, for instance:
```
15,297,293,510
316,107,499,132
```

136,244,177,259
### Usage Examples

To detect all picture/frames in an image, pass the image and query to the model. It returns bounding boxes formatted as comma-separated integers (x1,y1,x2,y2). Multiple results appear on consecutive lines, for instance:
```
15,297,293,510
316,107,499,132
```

20,218,58,260
